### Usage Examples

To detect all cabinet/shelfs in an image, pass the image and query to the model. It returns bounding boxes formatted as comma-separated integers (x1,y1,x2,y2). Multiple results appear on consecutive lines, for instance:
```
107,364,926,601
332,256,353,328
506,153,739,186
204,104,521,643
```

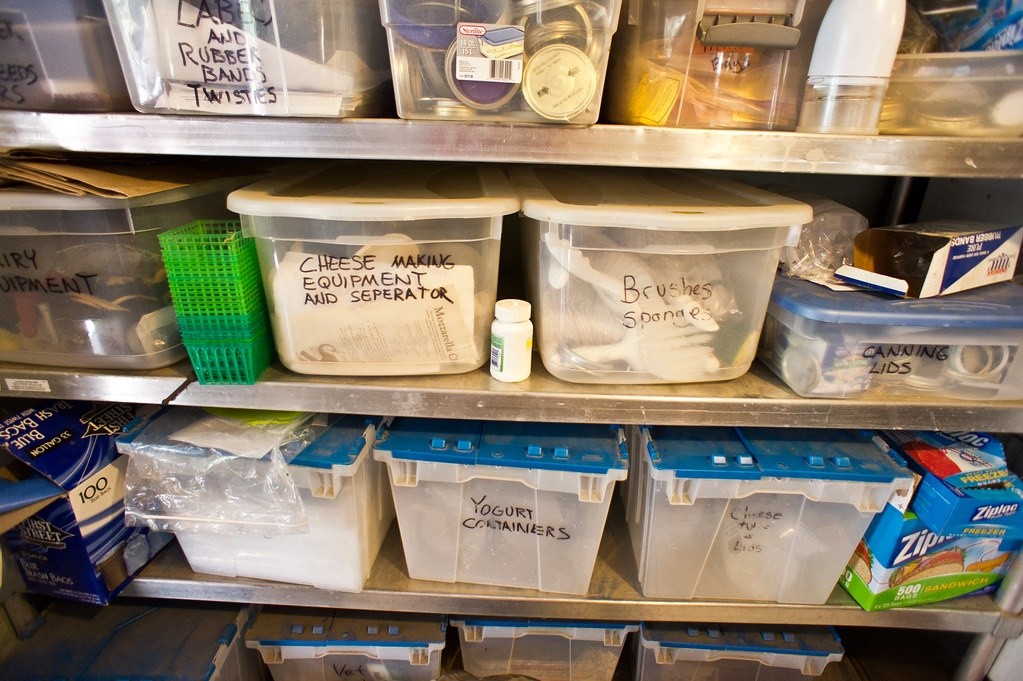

0,109,1023,681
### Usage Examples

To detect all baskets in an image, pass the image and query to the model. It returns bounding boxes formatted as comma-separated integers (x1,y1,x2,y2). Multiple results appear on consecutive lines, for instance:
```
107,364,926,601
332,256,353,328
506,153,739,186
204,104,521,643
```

157,220,271,387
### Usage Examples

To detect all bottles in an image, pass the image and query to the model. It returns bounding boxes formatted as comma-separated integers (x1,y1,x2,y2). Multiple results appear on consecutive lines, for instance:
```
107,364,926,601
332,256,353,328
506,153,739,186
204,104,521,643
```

490,299,533,382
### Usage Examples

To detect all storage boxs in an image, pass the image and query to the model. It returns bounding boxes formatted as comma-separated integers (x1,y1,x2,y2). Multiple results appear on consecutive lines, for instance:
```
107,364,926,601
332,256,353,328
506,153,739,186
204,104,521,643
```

0,0,1023,681
